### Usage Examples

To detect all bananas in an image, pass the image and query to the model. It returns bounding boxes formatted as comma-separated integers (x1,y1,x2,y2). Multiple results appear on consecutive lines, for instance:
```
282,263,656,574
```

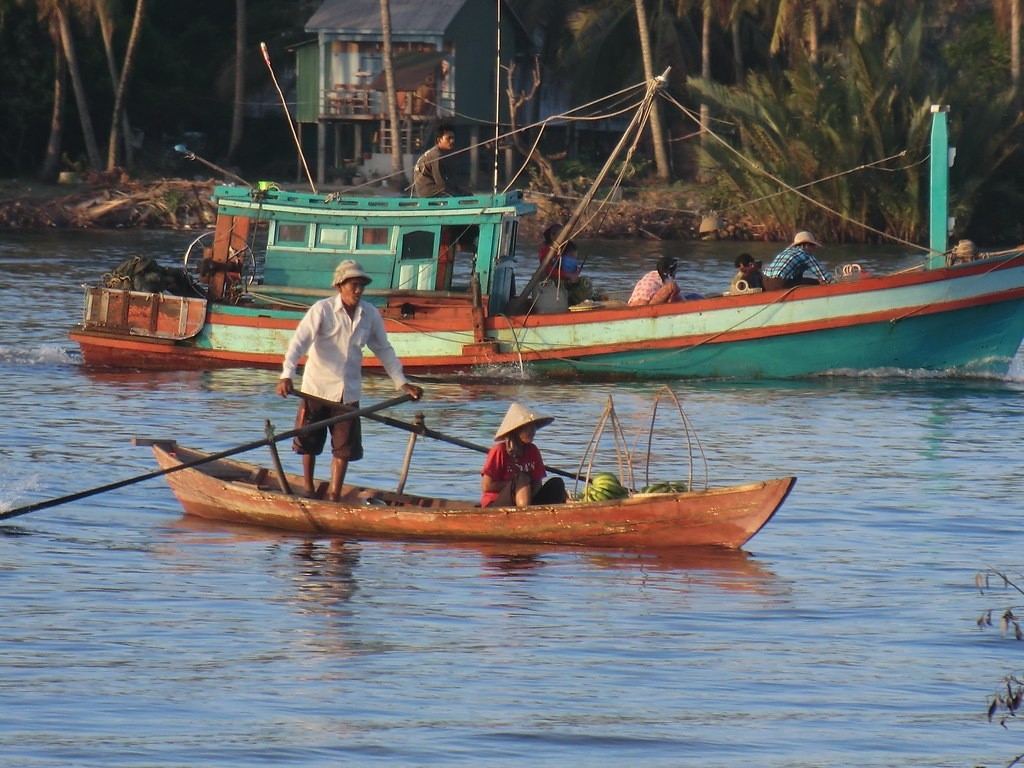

580,471,688,503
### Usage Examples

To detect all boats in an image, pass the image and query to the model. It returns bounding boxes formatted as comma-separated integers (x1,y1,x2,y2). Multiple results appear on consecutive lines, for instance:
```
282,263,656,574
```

132,436,797,549
71,67,1024,378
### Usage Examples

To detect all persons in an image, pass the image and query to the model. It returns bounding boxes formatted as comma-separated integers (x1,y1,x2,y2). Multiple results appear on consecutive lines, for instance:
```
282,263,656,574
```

275,260,423,502
762,231,837,292
539,224,594,304
414,125,473,198
481,402,565,507
736,254,764,289
627,256,679,304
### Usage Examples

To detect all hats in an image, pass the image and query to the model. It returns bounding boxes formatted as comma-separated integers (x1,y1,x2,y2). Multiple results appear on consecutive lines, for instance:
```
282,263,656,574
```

494,402,555,443
790,230,822,250
332,259,372,287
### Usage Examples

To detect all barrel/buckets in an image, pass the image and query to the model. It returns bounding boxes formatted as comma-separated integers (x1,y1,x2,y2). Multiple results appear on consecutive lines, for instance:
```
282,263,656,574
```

258,181,277,191
